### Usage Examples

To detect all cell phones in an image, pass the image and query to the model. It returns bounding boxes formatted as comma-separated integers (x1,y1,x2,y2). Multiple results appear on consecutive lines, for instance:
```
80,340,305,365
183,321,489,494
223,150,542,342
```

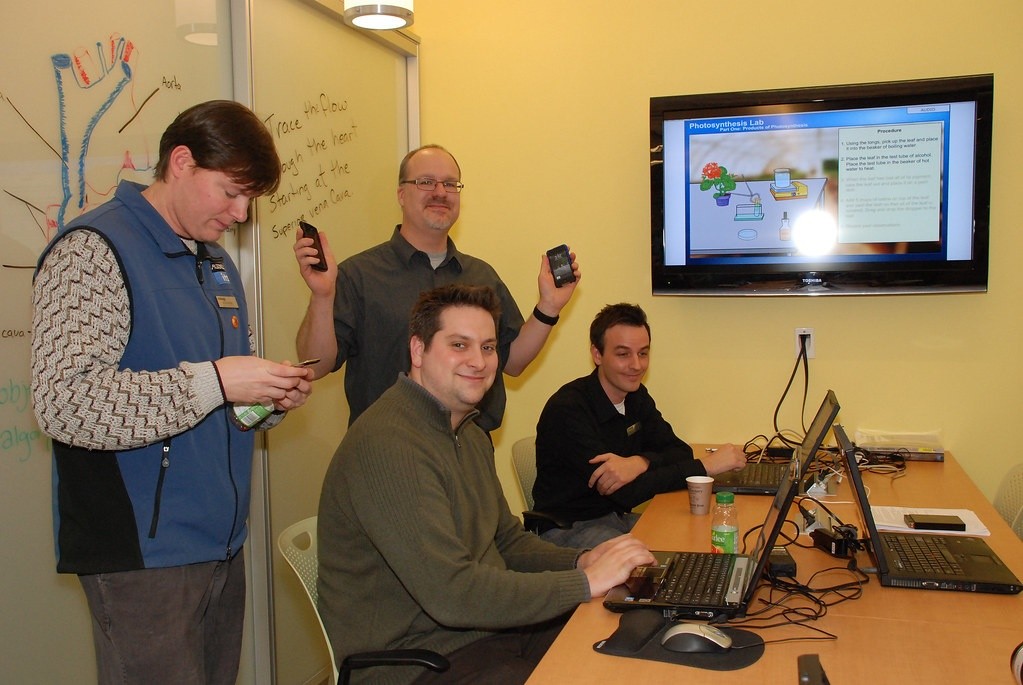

546,244,576,288
300,221,328,273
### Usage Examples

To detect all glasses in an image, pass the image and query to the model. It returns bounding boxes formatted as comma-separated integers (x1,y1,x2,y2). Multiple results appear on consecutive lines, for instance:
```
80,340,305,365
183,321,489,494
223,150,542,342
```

402,178,464,193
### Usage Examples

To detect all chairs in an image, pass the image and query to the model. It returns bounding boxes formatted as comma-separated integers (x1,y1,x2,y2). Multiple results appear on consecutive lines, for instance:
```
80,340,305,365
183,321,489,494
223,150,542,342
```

277,515,451,685
510,436,538,512
994,464,1023,543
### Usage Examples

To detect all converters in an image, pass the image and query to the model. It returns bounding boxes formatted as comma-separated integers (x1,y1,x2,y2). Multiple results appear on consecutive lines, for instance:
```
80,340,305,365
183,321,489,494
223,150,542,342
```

766,545,797,577
813,528,847,558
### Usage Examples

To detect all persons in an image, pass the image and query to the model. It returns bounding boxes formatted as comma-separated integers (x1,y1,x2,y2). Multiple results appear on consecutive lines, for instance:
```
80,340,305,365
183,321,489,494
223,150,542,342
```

317,283,658,685
32,100,314,685
522,302,747,549
293,145,581,451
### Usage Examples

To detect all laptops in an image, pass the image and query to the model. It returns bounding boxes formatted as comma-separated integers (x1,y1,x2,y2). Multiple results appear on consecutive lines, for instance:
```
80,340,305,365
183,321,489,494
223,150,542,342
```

602,441,803,620
831,420,1023,595
711,388,841,494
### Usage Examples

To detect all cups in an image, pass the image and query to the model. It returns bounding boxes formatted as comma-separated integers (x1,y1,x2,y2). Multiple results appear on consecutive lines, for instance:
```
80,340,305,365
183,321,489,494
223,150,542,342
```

686,476,714,515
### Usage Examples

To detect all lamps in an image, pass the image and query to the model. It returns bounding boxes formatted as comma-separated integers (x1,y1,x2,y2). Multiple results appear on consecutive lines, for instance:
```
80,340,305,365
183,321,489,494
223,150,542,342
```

174,0,217,46
343,0,414,31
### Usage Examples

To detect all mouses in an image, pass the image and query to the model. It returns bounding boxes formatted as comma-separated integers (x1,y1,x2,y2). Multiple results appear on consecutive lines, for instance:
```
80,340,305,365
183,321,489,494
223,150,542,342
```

660,625,734,654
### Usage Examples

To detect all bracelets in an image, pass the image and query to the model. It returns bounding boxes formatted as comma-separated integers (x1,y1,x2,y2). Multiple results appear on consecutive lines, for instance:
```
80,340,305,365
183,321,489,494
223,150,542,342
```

533,304,559,326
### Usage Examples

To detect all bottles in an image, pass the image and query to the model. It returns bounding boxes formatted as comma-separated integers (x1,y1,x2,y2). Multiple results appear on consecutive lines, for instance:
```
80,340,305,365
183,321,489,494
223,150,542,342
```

229,398,275,431
710,491,739,554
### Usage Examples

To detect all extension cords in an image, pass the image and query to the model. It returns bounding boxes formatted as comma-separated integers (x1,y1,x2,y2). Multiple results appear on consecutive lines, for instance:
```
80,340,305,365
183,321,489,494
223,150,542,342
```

805,472,829,499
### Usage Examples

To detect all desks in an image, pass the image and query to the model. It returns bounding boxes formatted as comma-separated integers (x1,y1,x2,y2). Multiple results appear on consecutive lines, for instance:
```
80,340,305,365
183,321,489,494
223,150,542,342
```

523,443,1023,685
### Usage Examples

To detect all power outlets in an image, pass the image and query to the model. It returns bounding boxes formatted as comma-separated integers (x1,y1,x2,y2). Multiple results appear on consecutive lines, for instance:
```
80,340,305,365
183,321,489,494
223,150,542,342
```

795,328,815,359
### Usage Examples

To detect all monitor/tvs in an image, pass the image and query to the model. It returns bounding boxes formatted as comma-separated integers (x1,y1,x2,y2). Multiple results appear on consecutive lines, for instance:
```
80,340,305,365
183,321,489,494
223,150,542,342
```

650,73,994,298
